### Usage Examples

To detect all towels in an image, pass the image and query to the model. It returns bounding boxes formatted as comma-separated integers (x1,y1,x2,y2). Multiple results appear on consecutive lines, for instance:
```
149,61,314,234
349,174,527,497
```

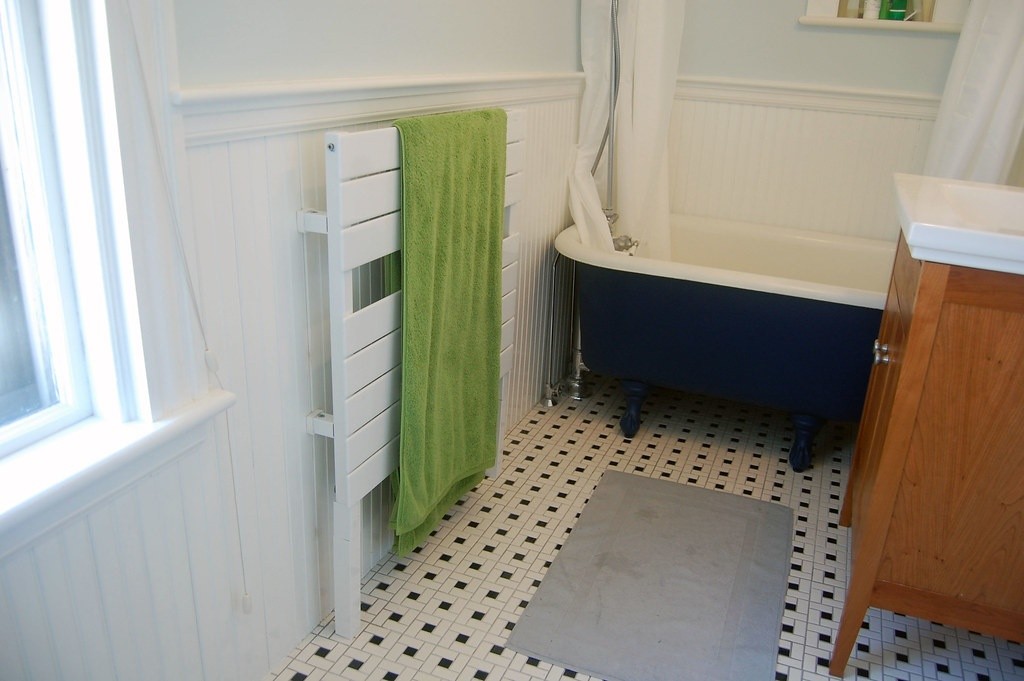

392,107,508,553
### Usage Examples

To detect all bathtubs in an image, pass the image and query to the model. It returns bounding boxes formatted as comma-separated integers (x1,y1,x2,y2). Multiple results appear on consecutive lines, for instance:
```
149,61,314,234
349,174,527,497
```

553,218,897,473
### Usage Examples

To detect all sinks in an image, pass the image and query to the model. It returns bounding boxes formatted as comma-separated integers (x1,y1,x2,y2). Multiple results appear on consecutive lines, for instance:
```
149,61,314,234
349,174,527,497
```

893,173,1024,276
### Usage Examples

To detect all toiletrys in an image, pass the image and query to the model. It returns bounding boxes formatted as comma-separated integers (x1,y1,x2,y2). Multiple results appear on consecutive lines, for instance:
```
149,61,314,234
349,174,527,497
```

837,0,936,22
